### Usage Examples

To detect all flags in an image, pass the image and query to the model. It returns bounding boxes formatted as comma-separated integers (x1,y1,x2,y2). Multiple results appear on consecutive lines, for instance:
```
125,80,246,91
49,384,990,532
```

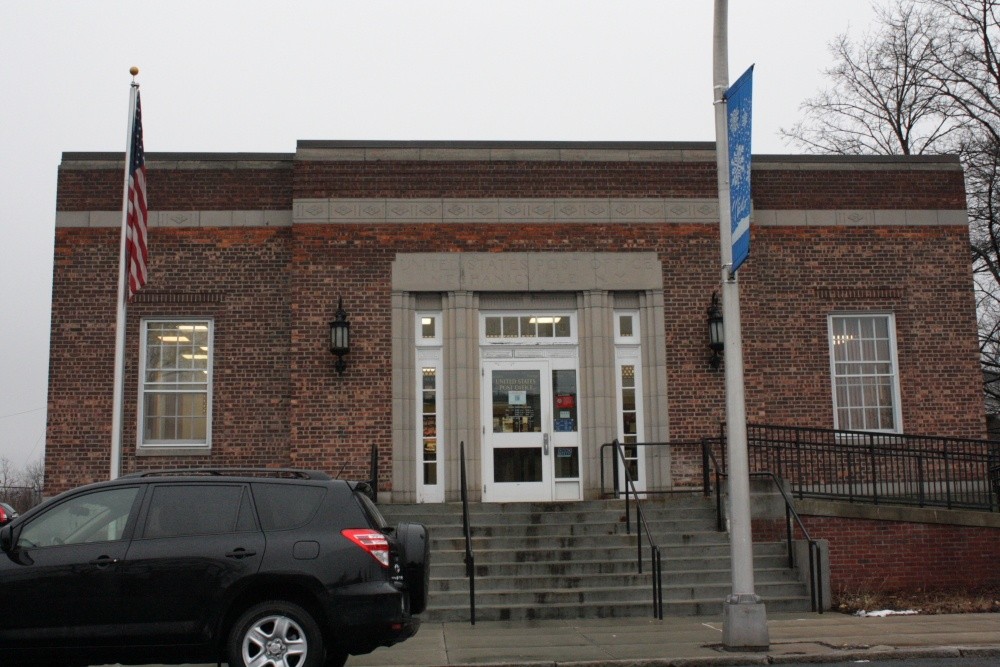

126,84,147,300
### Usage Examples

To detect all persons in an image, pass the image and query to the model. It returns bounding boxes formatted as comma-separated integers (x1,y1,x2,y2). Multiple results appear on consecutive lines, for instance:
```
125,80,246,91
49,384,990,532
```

512,394,535,482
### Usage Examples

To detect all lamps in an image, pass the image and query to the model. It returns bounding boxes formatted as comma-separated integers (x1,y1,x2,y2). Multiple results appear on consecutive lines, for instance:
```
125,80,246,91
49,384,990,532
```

329,298,349,374
707,290,724,366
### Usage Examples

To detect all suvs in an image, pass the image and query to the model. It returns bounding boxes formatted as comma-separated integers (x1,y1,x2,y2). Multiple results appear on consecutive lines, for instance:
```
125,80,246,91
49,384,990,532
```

0,465,432,667
